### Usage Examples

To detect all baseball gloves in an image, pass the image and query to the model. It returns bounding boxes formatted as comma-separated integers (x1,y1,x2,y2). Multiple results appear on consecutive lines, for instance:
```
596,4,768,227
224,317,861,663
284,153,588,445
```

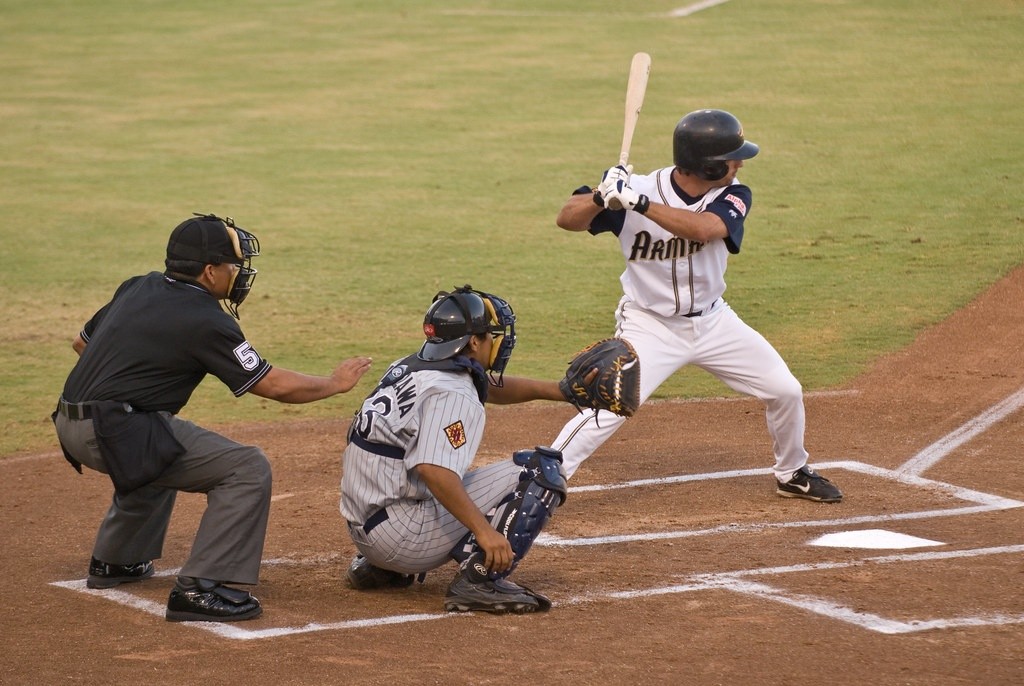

554,338,641,422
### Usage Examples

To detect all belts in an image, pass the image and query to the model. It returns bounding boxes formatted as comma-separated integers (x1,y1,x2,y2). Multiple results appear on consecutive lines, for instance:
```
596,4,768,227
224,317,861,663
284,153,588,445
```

346,508,388,535
680,300,717,317
58,397,92,419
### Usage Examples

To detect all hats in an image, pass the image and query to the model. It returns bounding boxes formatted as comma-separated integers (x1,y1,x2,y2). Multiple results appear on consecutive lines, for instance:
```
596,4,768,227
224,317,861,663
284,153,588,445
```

166,216,244,265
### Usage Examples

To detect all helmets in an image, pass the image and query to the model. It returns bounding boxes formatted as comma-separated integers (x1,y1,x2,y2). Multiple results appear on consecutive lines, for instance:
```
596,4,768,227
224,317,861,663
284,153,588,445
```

417,288,493,361
673,110,760,180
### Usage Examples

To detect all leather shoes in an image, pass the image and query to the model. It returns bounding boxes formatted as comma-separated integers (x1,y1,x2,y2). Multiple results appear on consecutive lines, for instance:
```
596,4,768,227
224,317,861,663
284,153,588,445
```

166,584,263,621
86,555,155,587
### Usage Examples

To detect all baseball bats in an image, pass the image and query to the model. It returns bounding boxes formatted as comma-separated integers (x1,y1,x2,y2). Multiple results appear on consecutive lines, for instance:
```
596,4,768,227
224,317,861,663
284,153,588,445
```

608,52,652,211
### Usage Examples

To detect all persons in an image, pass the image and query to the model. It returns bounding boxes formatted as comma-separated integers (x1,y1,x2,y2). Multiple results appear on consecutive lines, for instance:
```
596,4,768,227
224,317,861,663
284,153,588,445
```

51,212,374,622
340,282,641,613
551,108,845,504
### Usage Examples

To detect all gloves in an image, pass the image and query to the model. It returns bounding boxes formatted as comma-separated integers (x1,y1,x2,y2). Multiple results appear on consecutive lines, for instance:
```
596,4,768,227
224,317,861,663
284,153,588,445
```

602,179,650,214
593,164,633,207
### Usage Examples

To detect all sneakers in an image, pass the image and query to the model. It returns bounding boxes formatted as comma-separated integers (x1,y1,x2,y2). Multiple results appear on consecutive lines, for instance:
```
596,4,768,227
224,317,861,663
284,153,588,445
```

776,465,842,501
345,554,414,589
443,571,539,613
